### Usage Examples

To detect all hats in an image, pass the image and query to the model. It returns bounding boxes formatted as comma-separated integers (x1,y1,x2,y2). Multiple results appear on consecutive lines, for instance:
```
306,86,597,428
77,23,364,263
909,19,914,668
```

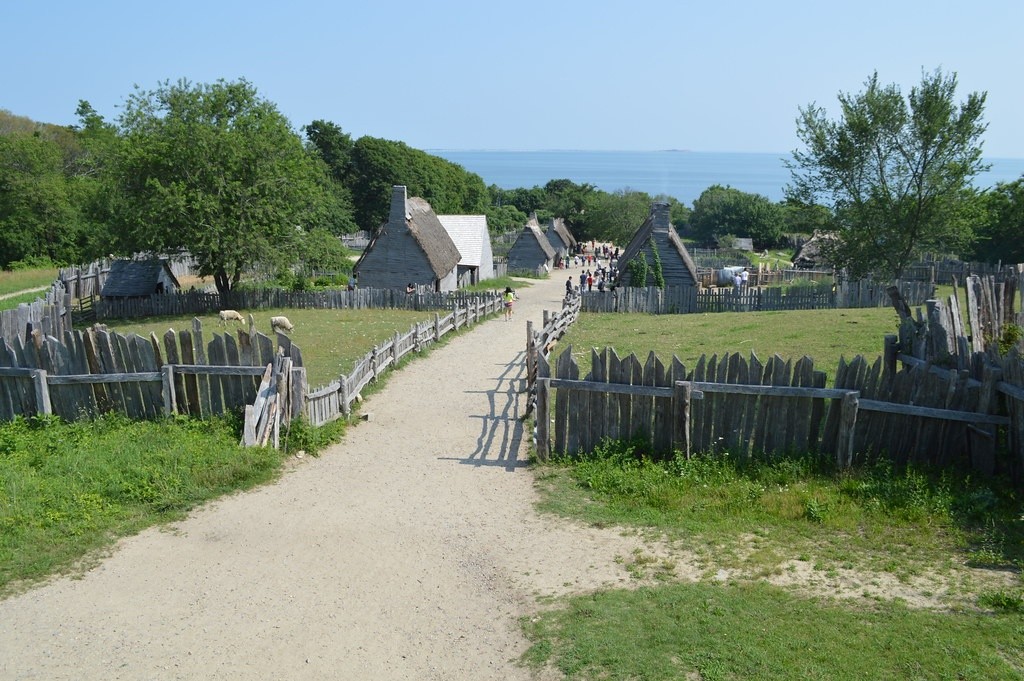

504,287,515,293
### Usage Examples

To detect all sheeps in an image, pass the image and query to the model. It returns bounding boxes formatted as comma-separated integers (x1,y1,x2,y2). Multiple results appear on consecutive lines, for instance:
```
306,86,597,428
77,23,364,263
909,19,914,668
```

217,310,296,335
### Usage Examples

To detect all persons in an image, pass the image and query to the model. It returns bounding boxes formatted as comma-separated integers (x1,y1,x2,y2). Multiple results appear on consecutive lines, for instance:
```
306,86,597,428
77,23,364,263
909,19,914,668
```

735,268,749,284
406,282,415,293
348,275,356,291
504,287,513,321
559,242,619,296
